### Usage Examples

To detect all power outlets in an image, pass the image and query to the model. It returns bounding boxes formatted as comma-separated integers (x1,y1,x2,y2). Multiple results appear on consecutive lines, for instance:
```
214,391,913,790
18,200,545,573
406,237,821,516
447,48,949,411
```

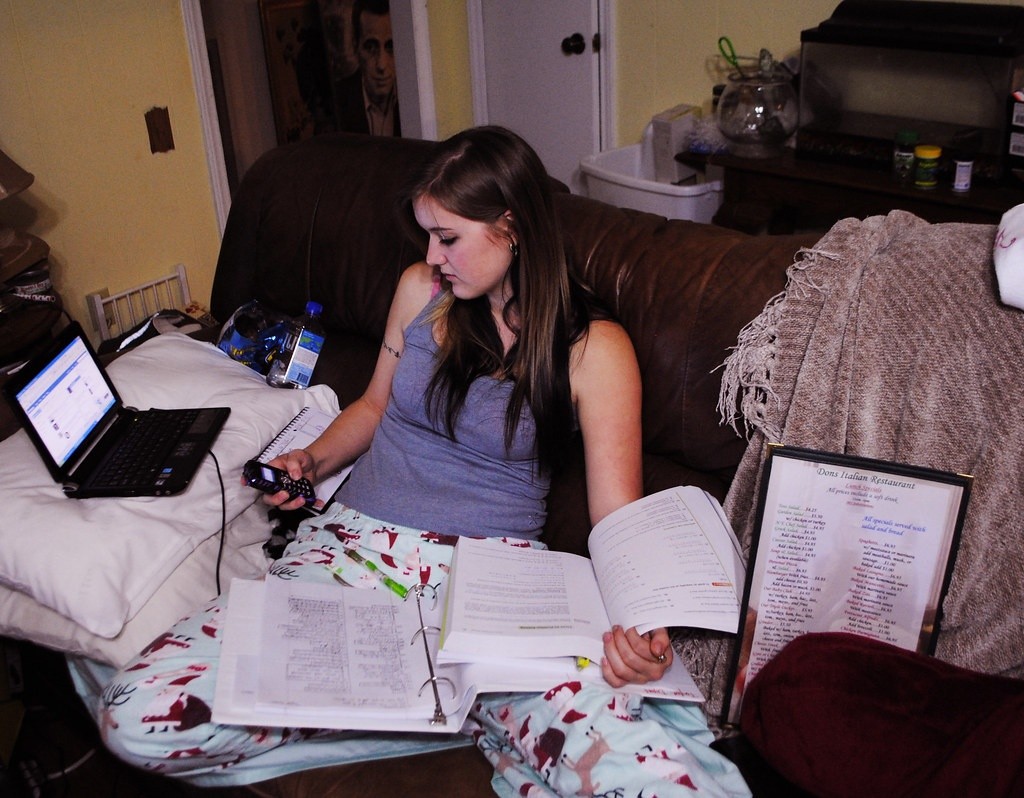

86,288,116,333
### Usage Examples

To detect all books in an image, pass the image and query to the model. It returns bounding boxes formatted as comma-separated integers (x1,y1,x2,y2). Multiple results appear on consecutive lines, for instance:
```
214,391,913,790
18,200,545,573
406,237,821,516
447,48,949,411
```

209,570,708,736
430,483,746,672
254,406,356,516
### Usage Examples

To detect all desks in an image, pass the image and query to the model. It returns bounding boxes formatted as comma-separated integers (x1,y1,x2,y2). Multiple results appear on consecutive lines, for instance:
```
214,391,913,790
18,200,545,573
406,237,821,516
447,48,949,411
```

675,145,1024,236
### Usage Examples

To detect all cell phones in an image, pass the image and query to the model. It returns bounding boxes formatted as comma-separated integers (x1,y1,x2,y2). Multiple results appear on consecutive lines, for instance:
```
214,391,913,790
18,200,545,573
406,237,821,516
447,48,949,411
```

246,460,317,508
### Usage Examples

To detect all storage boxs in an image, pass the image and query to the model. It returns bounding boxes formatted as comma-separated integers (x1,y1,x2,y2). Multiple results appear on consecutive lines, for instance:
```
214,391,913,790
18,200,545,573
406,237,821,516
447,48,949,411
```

267,326,325,388
579,145,725,223
652,104,703,184
796,27,1024,183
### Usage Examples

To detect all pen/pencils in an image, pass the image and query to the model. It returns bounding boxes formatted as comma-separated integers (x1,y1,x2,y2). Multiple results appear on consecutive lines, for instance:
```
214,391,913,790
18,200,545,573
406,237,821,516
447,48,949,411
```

342,545,409,599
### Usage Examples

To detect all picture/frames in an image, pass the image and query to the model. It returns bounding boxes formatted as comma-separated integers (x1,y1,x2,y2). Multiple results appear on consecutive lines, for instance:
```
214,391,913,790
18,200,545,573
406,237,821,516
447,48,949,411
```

258,0,401,146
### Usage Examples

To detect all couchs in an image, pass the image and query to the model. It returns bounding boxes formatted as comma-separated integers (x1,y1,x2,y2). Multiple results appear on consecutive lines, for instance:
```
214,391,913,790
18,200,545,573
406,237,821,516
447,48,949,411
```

1,136,1024,798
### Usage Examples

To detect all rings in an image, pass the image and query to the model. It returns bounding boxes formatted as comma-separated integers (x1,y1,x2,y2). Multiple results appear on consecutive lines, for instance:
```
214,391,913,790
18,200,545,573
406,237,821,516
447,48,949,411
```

658,654,668,664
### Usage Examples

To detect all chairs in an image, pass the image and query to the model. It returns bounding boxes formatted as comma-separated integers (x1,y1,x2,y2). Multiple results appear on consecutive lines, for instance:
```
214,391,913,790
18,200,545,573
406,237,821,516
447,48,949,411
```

91,264,191,342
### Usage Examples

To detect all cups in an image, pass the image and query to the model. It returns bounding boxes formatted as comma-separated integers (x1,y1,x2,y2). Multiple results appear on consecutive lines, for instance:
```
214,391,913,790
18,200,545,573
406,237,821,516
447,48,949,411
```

715,71,801,159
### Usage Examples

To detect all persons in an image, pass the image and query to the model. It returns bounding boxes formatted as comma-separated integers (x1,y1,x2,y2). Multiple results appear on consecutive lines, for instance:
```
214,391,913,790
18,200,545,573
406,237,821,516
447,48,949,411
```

91,122,760,798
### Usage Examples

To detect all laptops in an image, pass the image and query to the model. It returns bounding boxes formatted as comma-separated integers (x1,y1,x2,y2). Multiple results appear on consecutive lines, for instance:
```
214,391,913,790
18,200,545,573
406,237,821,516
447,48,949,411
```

0,321,232,500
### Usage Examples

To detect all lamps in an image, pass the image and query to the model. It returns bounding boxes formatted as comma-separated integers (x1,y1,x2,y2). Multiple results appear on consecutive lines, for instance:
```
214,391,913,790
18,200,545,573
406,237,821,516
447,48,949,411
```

0,150,34,250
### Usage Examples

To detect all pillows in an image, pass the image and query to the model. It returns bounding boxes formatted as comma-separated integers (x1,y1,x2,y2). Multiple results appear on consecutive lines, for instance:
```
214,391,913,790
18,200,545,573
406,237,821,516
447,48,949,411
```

0,333,340,669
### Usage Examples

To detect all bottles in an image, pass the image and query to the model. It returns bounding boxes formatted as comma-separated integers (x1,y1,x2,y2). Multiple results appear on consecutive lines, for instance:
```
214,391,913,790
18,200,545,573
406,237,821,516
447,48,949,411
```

267,301,326,390
220,298,271,365
893,129,920,181
248,318,290,376
